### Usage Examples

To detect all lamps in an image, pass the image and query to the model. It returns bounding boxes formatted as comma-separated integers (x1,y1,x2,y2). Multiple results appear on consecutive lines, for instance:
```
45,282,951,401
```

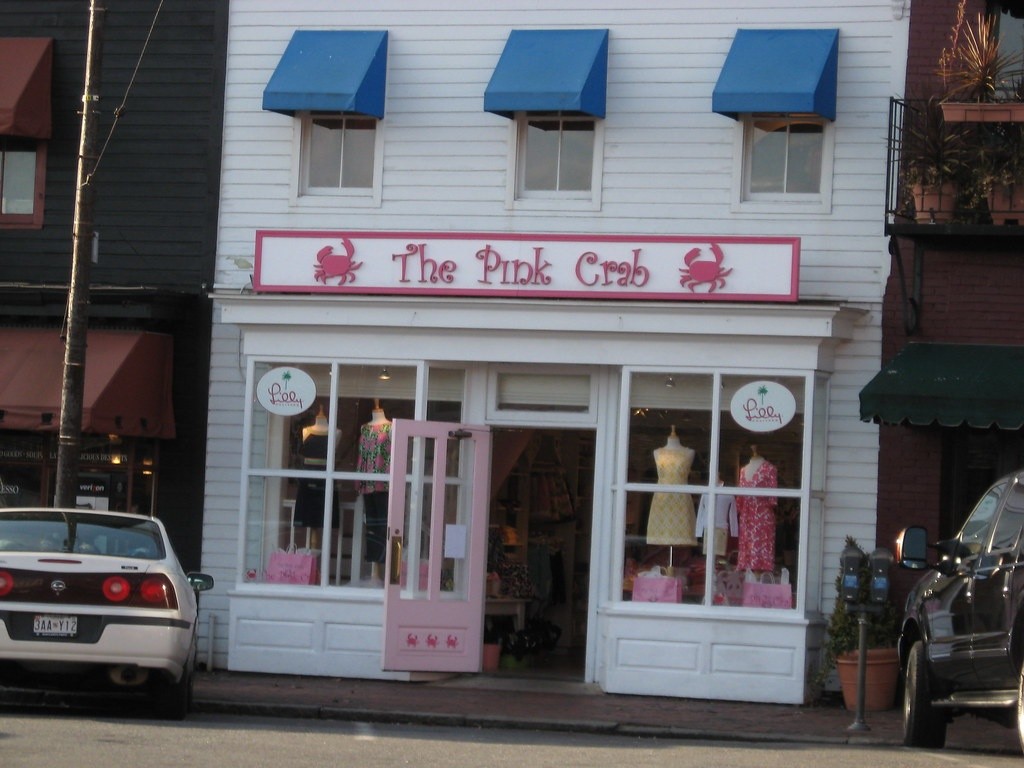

665,374,676,389
378,366,392,380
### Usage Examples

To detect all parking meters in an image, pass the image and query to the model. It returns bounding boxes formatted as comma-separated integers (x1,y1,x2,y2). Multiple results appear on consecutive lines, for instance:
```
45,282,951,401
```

838,545,896,732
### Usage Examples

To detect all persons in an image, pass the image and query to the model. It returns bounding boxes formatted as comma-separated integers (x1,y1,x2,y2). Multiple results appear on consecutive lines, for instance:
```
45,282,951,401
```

652,436,695,547
301,411,342,528
354,409,393,564
739,455,778,573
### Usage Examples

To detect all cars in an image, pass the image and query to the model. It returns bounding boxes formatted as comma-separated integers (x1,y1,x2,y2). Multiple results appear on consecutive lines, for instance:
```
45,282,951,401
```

1,505,215,722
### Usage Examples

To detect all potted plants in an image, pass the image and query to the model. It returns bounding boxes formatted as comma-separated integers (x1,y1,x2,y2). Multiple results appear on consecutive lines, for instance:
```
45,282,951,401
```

975,123,1024,225
899,84,959,224
810,534,899,712
939,0,1024,123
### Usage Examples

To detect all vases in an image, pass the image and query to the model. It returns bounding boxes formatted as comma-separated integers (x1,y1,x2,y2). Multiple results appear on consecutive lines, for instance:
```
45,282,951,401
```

483,645,503,671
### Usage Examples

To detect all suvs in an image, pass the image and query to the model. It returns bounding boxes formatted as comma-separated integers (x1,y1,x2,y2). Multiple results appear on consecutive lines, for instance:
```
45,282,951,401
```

897,470,1024,755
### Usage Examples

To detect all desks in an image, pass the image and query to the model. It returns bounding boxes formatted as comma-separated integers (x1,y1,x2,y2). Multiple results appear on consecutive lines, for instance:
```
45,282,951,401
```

485,597,530,633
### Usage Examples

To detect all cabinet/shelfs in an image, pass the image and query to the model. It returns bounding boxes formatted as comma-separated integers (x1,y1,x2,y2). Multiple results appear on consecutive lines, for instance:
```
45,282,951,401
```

488,435,595,647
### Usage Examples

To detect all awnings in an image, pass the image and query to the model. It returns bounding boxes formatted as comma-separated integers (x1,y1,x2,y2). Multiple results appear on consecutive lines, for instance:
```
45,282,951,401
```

712,29,840,119
483,31,608,120
859,344,1024,432
0,36,52,141
1,325,176,445
264,31,387,119
